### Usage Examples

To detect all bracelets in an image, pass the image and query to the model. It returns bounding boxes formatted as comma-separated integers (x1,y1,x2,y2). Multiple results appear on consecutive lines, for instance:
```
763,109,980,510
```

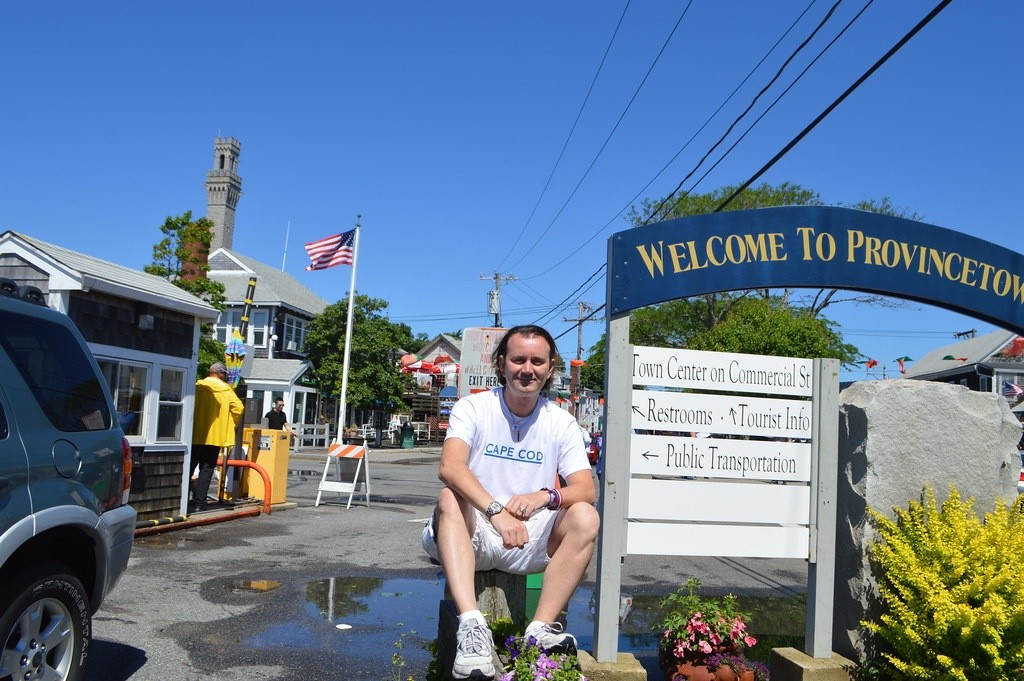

541,487,562,510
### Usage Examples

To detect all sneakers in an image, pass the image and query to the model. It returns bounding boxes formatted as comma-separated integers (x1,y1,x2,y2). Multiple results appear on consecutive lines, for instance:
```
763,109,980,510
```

452,610,496,681
522,620,579,657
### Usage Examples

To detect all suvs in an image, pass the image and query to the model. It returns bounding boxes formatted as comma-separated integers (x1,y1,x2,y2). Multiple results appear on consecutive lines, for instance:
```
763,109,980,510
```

0,292,138,681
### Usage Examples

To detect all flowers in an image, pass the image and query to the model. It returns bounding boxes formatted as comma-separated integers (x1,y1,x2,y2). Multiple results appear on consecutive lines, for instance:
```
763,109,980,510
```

650,577,771,681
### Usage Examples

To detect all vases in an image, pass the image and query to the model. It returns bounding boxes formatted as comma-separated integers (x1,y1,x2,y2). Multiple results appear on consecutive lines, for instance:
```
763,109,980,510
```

667,643,756,681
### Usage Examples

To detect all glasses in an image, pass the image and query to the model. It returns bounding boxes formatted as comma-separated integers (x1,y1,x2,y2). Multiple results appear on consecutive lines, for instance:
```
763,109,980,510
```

278,404,283,406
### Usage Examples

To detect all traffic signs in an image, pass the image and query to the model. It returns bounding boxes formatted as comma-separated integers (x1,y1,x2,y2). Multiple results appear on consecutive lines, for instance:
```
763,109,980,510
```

630,433,810,483
631,345,814,397
632,389,812,441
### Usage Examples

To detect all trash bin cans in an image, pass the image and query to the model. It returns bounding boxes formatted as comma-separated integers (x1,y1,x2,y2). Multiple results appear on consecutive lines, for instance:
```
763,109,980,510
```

400,426,415,449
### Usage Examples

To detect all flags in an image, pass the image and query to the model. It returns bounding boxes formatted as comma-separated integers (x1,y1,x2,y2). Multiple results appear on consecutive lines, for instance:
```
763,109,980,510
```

303,228,356,271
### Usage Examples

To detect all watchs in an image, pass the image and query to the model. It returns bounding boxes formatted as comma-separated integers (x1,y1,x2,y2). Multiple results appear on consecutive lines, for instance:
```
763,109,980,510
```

486,502,503,521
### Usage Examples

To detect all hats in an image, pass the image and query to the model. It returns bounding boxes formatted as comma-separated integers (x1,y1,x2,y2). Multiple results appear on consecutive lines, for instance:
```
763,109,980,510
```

210,363,230,374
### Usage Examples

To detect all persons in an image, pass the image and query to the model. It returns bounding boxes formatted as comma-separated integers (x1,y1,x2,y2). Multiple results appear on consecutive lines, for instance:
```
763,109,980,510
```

187,362,245,512
265,398,298,438
421,325,599,680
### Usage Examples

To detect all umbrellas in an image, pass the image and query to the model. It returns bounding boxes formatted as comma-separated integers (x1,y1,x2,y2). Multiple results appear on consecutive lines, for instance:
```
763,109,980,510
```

400,353,460,391
222,326,247,395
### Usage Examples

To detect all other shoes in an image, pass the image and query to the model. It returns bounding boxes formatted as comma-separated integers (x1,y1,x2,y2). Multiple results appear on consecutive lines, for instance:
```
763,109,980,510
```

194,504,205,512
